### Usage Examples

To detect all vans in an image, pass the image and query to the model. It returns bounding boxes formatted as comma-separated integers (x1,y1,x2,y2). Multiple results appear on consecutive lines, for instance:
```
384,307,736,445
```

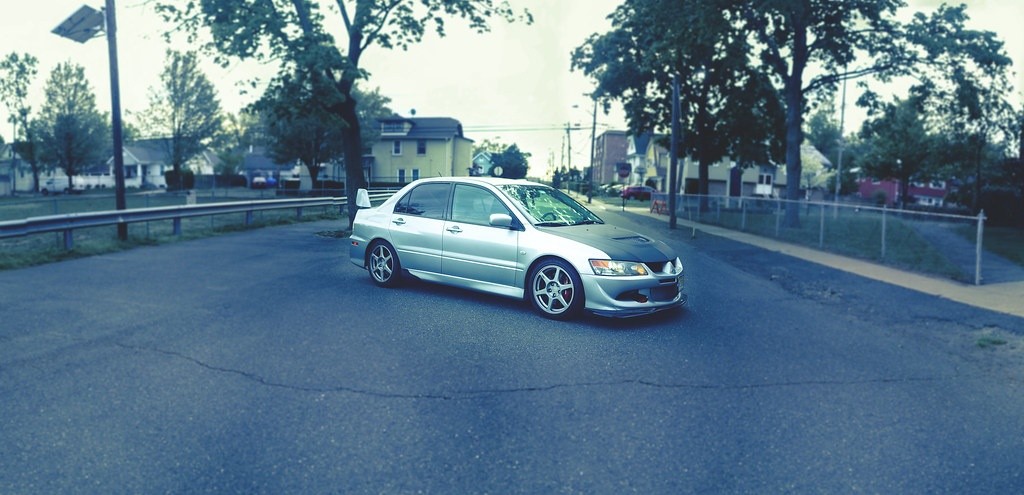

41,175,85,196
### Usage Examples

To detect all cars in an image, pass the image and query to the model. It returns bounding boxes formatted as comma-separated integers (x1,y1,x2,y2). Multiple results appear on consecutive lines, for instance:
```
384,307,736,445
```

347,174,689,323
597,183,660,200
252,177,267,189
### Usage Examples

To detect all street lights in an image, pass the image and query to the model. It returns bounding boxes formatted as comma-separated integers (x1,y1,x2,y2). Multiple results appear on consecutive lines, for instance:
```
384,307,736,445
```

571,105,596,203
